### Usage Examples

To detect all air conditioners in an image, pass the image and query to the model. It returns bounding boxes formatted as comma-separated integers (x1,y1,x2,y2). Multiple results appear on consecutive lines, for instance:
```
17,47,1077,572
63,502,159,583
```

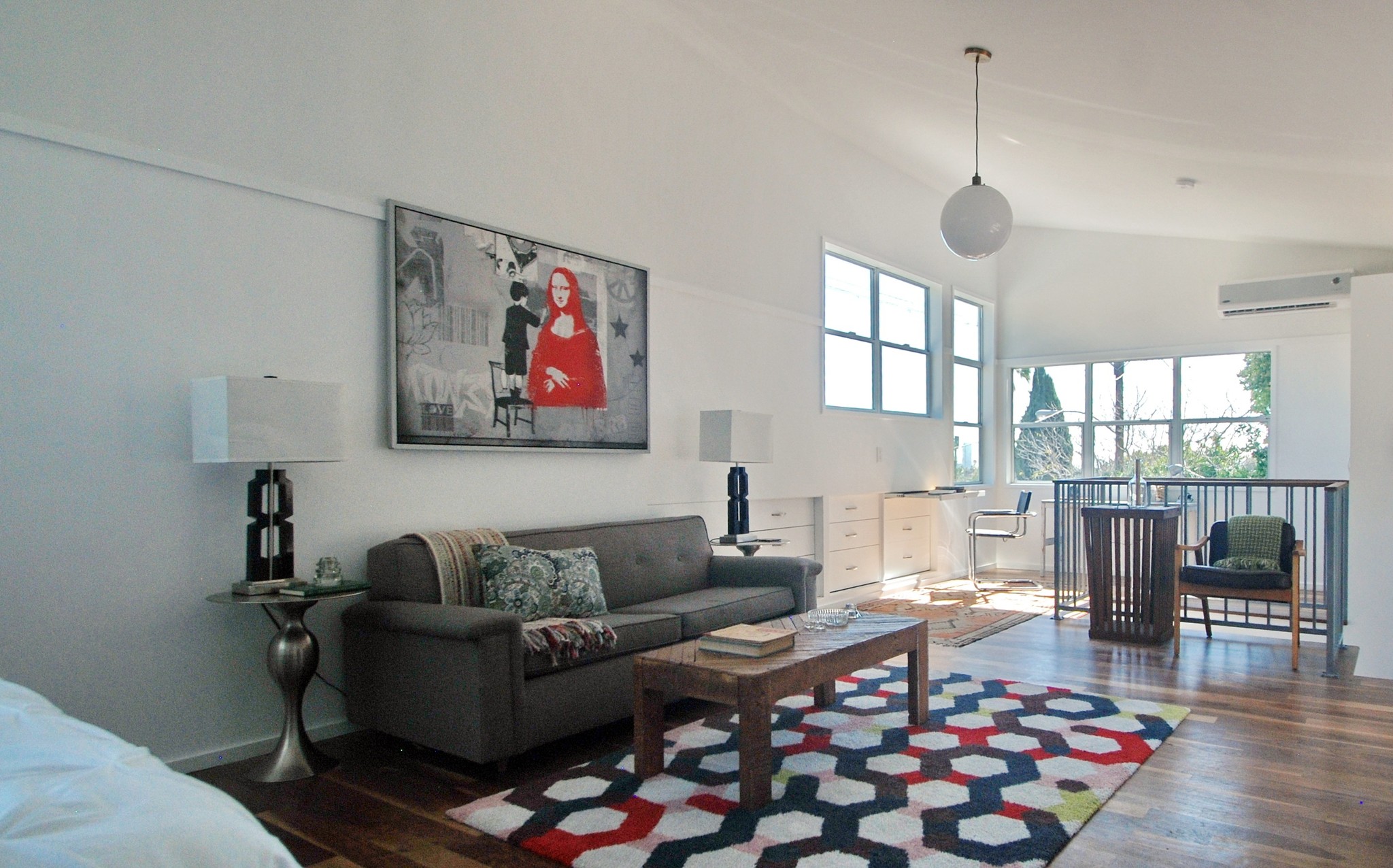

1216,272,1355,317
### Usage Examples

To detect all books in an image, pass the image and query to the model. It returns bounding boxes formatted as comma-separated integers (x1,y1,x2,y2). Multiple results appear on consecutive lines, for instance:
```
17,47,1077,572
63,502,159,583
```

698,622,801,658
279,578,365,596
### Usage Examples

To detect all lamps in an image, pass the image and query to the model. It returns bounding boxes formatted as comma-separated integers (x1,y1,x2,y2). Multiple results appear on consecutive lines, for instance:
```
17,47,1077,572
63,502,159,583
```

698,410,775,544
191,375,346,596
939,45,1013,260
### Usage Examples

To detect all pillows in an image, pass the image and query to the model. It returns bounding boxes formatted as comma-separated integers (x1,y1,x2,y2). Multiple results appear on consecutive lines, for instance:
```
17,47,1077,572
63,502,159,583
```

473,543,607,623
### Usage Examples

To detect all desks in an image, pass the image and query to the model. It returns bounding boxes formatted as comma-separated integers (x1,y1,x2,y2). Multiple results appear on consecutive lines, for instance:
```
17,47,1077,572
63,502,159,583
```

1041,498,1197,578
205,588,373,783
1082,503,1185,646
710,536,791,558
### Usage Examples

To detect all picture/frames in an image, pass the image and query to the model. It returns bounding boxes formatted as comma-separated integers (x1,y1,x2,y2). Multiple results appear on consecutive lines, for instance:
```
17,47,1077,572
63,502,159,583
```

385,198,652,457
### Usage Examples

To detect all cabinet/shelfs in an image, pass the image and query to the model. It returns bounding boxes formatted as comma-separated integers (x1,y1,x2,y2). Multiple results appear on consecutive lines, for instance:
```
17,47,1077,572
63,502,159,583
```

653,495,936,611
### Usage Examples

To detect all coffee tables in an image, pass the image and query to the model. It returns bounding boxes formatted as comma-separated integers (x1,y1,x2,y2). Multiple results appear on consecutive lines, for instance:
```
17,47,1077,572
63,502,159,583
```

629,609,931,811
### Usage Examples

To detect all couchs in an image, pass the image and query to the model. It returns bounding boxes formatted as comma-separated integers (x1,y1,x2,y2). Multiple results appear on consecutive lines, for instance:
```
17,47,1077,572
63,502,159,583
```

0,678,302,867
343,515,824,772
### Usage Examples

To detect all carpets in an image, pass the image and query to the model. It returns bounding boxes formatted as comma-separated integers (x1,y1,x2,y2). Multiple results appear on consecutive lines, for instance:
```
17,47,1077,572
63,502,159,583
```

841,579,1084,647
446,656,1192,867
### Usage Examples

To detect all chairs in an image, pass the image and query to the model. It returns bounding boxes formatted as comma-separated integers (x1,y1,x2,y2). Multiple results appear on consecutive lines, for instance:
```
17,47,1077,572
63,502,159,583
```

1173,516,1306,671
965,489,1043,591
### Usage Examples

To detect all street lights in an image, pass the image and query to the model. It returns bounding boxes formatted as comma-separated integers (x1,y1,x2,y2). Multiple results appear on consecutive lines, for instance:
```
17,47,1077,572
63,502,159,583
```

1034,409,1124,472
